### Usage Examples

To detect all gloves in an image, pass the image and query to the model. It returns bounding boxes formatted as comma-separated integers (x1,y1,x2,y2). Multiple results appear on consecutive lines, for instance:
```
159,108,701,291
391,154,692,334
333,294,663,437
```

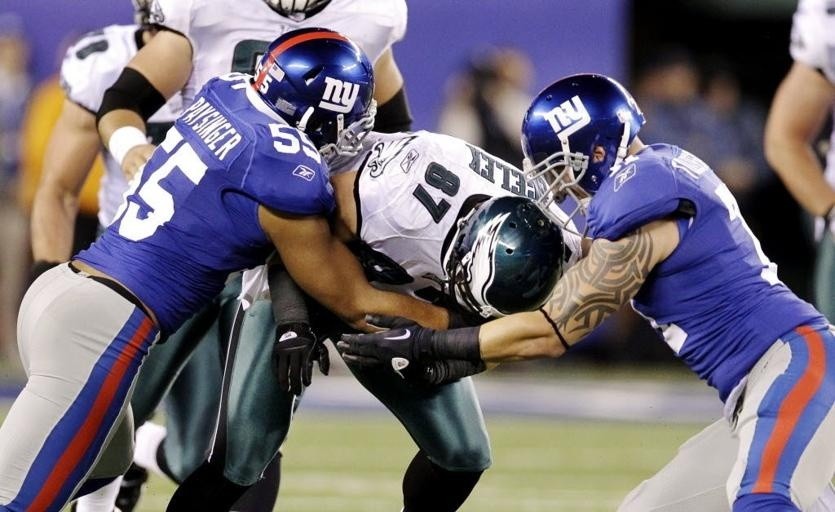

333,312,440,364
266,318,334,395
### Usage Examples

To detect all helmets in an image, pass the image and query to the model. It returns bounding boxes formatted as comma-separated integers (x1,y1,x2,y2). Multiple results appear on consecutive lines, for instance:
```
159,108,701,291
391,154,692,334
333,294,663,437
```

249,23,376,174
514,71,646,241
443,190,567,324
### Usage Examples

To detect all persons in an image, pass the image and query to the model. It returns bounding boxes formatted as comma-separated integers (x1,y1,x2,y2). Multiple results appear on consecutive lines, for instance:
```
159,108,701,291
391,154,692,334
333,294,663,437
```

167,128,580,510
74,0,416,511
335,72,835,510
29,0,226,511
1,27,776,371
764,0,835,329
1,26,497,511
